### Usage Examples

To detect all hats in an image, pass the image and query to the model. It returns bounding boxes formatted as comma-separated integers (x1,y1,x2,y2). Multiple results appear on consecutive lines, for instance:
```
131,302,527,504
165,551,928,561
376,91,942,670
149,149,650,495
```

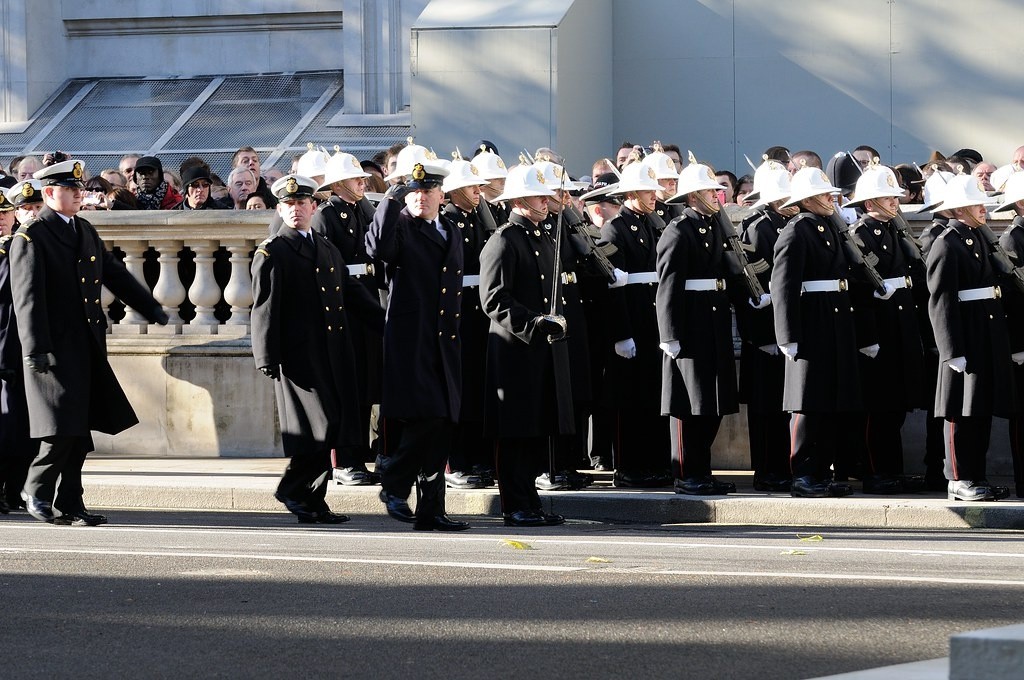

133,156,164,184
182,166,213,193
271,174,319,203
405,163,450,189
0,160,86,213
578,182,622,207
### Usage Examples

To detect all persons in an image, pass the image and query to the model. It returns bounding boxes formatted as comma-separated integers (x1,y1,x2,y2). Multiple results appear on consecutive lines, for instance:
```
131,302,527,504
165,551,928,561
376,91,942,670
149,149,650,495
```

0,135,1024,530
9,161,170,527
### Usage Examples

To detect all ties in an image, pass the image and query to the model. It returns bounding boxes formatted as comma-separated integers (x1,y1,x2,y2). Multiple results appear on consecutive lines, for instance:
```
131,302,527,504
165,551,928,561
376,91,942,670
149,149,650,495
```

431,221,437,229
69,218,75,233
306,233,315,246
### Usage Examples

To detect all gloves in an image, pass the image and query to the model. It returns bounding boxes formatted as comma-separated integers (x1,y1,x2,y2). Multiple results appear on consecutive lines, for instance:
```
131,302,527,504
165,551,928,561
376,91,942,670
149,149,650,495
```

382,181,414,201
748,294,771,310
613,336,1024,373
259,363,280,382
24,353,50,375
152,308,169,326
539,319,563,337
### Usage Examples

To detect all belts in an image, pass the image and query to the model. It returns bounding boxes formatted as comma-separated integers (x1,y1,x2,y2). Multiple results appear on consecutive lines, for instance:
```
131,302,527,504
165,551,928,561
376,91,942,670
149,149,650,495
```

627,271,1003,302
560,272,578,285
461,275,481,287
345,263,376,276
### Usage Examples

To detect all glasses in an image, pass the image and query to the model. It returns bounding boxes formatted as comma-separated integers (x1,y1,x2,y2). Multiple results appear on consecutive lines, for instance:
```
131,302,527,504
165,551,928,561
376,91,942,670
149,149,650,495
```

84,187,106,192
189,182,210,188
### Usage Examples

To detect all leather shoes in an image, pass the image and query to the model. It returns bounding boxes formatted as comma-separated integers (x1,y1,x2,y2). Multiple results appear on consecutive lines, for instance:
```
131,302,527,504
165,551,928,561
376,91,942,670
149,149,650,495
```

0,479,22,513
413,511,470,530
503,507,564,525
332,453,1010,500
274,487,350,522
54,493,107,524
20,488,54,523
378,485,415,523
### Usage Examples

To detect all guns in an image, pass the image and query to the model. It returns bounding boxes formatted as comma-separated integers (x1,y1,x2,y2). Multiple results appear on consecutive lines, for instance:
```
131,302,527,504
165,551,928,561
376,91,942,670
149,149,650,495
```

312,138,1024,312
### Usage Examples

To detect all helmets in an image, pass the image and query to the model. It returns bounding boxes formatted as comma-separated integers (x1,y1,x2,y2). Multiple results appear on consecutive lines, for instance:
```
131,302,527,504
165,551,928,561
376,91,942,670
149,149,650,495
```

291,136,1024,214
